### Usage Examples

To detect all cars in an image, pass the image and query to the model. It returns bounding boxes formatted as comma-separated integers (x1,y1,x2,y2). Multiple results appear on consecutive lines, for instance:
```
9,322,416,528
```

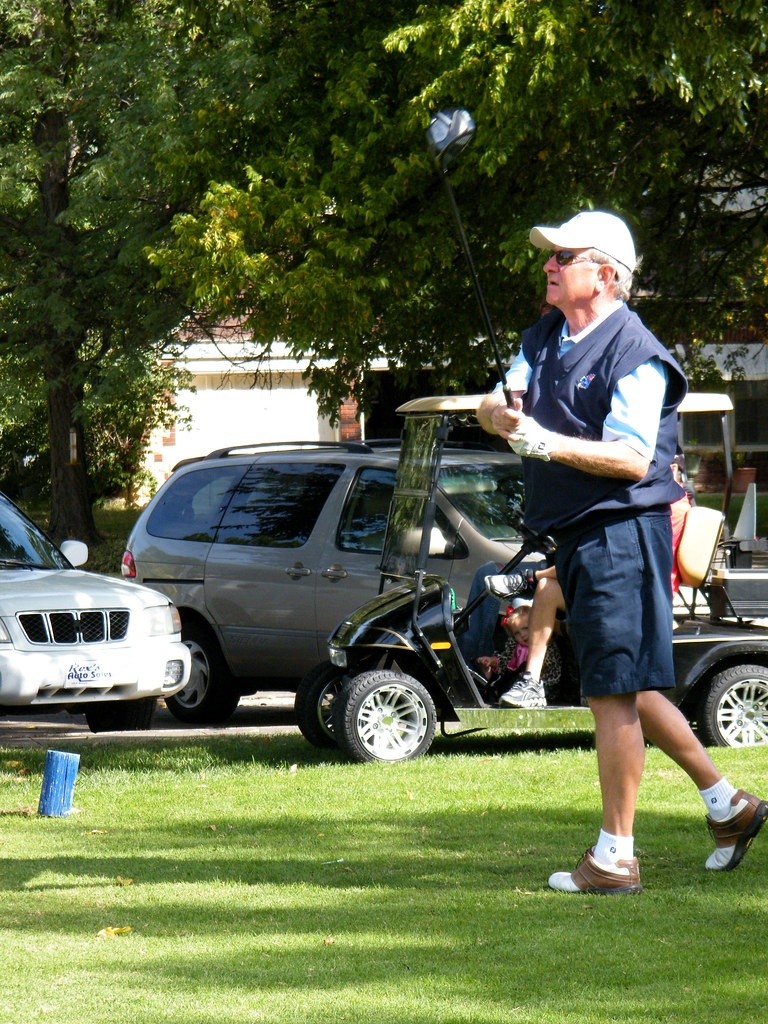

1,490,196,734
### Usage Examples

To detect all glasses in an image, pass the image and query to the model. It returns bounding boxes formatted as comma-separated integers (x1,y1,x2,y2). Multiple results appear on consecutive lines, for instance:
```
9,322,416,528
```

548,250,604,266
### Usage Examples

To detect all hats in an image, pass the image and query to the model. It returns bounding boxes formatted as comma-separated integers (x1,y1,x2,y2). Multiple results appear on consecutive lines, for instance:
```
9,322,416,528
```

530,211,636,274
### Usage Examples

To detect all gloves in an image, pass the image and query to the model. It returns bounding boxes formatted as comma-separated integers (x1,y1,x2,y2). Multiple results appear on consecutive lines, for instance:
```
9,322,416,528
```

507,416,555,461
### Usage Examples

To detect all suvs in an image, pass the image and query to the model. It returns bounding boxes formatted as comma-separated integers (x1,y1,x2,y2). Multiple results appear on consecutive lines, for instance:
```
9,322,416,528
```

121,434,575,728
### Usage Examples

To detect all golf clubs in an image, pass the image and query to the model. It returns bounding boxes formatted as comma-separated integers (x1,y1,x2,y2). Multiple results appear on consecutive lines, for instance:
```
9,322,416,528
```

424,103,516,412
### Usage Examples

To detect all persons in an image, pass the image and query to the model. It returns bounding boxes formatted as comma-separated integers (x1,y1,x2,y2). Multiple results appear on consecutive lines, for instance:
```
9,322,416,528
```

476,450,696,708
477,212,768,894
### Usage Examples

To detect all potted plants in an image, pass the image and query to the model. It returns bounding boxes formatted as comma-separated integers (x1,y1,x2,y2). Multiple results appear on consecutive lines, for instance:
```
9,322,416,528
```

720,445,755,494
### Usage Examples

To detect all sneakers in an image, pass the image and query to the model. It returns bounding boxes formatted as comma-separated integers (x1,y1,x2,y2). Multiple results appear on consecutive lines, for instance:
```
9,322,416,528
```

704,789,768,870
484,568,537,604
498,672,546,707
548,844,642,894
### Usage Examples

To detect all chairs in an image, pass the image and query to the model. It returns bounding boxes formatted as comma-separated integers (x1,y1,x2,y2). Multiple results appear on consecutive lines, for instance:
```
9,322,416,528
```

672,507,726,635
360,488,392,551
